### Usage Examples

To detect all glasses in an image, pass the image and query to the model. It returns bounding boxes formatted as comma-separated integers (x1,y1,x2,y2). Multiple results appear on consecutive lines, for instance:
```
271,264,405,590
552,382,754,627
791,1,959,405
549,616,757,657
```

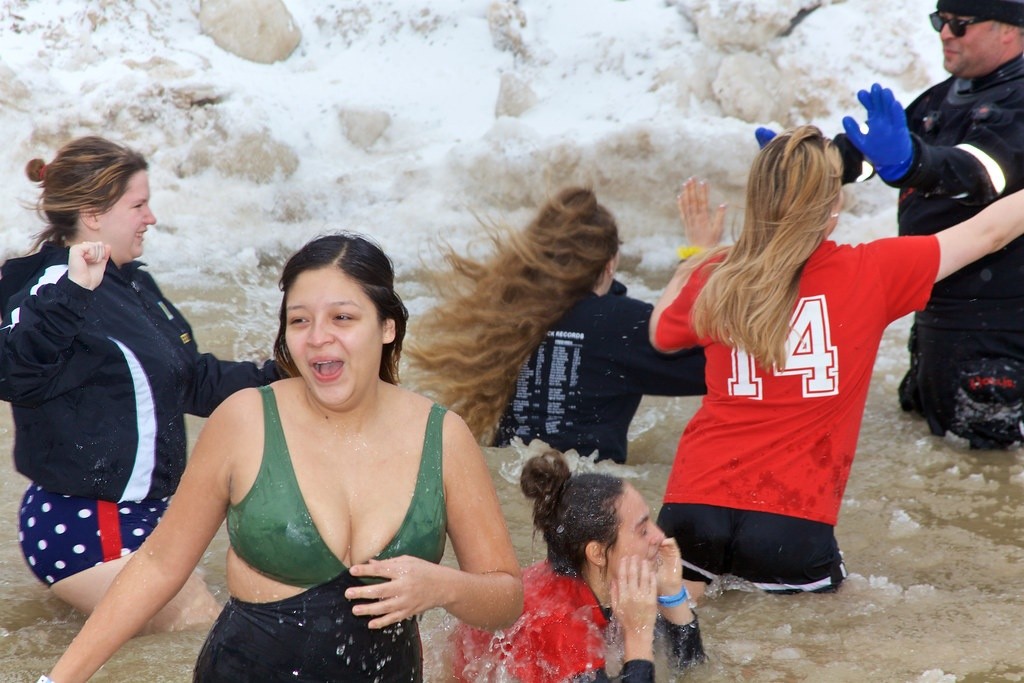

930,12,989,38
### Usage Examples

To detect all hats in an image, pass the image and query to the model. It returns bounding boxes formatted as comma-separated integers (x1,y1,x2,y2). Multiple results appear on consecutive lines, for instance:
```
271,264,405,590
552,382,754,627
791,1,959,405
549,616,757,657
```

936,0,1023,29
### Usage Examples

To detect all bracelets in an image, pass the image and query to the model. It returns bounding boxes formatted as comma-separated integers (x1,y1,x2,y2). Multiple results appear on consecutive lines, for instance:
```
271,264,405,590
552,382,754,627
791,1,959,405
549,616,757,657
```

657,585,689,608
36,675,55,683
678,245,707,261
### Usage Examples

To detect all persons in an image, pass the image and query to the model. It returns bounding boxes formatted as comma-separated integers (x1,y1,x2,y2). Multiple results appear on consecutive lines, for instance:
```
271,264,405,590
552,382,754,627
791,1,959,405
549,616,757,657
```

449,452,706,683
650,126,1023,599
757,0,1024,454
405,179,709,465
38,233,525,682
0,138,300,642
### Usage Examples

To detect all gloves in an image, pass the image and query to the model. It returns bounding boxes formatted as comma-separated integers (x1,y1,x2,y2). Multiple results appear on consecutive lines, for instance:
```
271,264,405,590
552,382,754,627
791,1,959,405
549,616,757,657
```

843,84,917,182
756,127,775,147
